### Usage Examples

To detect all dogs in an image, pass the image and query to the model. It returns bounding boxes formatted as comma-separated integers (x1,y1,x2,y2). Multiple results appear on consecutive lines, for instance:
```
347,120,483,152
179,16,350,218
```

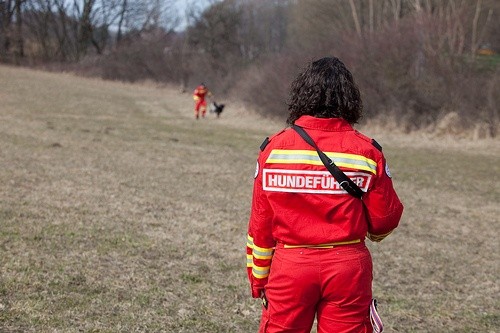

209,101,225,118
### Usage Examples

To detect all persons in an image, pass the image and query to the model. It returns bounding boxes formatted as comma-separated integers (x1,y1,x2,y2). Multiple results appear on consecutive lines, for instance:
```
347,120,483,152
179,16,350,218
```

246,56,403,333
192,82,214,120
